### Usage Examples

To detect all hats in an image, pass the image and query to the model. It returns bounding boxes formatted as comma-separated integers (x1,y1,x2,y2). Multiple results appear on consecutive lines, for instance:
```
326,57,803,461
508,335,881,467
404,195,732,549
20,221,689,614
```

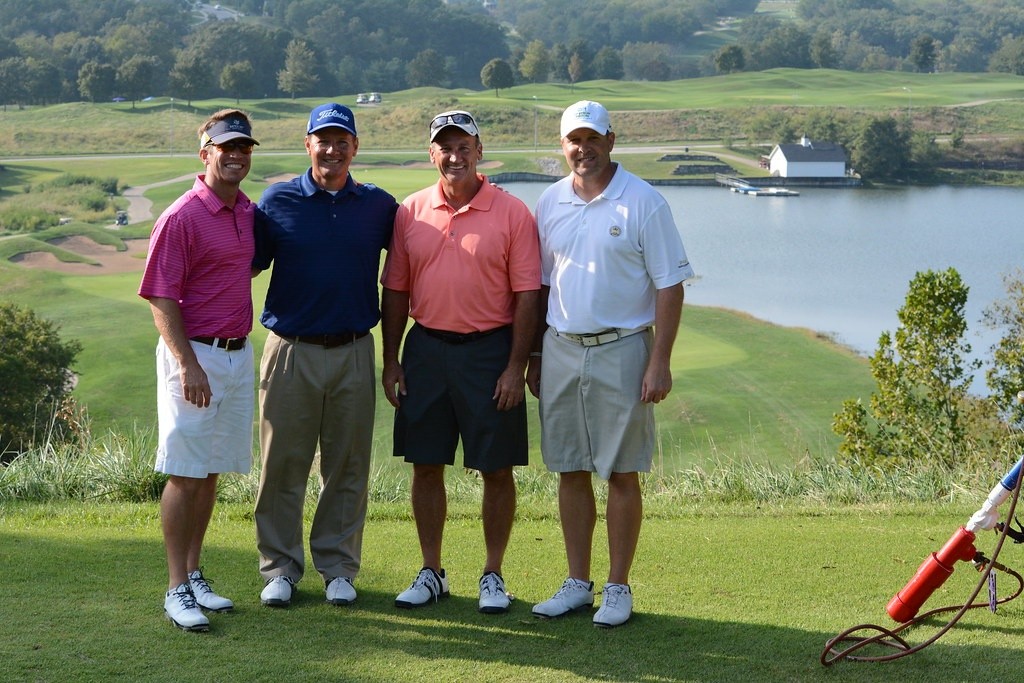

560,100,611,140
430,110,481,143
201,118,259,150
307,102,356,137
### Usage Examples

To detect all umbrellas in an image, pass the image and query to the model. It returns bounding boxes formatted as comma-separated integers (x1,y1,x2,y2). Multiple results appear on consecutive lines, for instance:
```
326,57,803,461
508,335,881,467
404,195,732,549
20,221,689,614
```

113,97,125,100
143,96,154,100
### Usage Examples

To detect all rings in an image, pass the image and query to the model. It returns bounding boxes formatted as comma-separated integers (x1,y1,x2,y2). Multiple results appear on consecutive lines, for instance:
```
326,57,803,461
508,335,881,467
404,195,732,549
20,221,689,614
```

507,399,514,403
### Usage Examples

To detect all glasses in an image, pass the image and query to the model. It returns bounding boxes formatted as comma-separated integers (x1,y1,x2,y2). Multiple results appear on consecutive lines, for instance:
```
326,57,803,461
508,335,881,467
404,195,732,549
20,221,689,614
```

205,142,253,154
430,113,479,138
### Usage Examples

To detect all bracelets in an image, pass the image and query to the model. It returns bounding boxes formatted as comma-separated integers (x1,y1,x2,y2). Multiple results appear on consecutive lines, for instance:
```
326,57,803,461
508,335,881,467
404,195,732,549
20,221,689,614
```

528,352,542,356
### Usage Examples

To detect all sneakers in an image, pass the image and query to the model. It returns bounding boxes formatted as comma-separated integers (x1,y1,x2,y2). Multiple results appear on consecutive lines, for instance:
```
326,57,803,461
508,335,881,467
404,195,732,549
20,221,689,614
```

592,582,633,627
188,566,234,611
260,575,294,606
164,582,209,631
478,570,510,614
325,577,358,606
394,566,450,608
531,576,594,618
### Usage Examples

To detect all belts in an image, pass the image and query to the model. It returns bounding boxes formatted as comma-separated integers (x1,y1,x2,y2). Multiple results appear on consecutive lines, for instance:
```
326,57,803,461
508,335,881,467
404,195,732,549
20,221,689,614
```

549,323,653,347
415,321,509,346
190,336,247,350
279,329,370,350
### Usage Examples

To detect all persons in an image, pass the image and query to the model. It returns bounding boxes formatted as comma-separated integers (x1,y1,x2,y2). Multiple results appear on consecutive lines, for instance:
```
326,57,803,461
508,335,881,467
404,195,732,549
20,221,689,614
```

380,110,542,613
525,100,694,628
250,103,399,607
139,109,261,630
